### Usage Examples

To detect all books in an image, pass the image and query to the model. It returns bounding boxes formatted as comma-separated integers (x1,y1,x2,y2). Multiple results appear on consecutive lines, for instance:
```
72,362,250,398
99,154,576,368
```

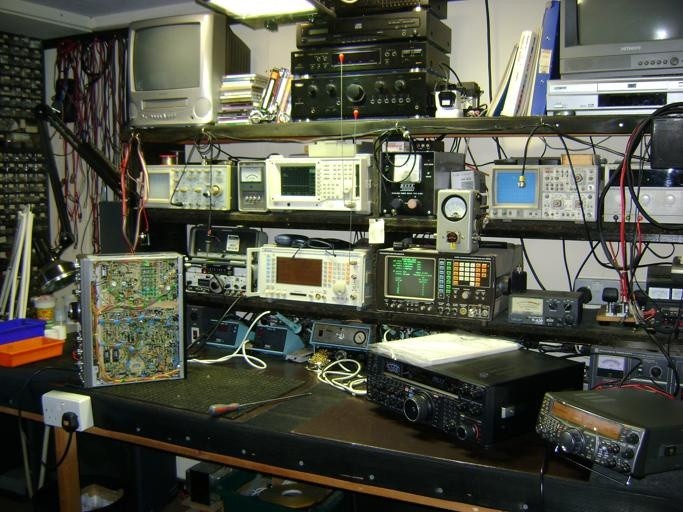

214,67,294,125
484,0,560,117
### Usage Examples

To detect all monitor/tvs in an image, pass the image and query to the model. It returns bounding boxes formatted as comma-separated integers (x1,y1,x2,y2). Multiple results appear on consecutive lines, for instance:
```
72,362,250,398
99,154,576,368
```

560,0,683,80
127,12,250,128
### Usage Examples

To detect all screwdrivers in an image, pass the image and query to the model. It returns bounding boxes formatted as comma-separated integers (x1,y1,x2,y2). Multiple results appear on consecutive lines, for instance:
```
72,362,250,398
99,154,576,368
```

209,393,313,417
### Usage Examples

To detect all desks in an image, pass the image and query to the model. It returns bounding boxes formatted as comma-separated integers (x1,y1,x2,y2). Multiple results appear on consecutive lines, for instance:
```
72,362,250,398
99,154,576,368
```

0,317,682,512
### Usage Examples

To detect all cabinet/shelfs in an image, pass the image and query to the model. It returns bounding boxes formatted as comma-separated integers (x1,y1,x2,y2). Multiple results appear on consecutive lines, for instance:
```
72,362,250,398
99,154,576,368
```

122,107,683,348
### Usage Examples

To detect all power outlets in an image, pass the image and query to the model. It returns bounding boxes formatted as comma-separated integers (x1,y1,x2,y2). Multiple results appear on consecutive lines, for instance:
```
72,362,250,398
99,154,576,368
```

40,391,94,432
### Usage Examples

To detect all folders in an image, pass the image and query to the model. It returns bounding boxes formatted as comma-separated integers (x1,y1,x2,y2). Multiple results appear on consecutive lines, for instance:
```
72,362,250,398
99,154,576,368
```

532,0,559,115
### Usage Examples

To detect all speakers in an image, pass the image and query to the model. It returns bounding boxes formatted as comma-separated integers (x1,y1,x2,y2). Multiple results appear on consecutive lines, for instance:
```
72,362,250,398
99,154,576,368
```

436,189,480,253
